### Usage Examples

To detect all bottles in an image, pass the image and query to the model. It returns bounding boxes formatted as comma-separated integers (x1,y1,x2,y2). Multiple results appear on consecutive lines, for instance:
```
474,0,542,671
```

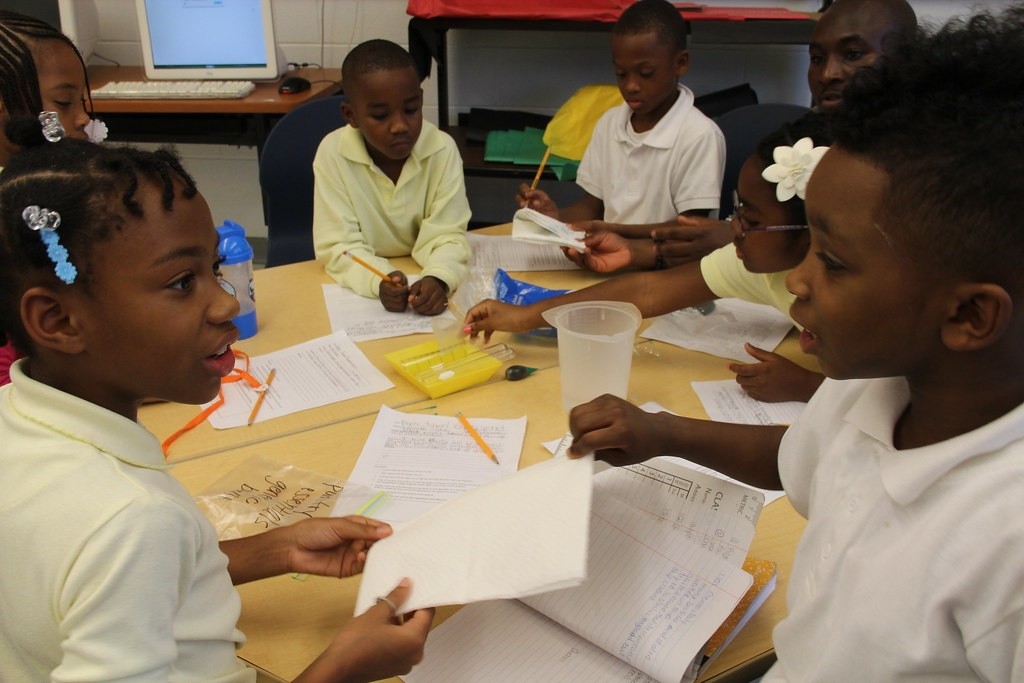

211,217,258,341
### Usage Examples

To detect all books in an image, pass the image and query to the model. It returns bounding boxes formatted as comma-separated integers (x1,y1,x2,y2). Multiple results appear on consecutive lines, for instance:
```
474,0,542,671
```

392,435,778,683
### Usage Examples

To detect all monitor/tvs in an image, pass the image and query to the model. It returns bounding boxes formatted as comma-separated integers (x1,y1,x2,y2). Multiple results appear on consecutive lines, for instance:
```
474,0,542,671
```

135,0,287,82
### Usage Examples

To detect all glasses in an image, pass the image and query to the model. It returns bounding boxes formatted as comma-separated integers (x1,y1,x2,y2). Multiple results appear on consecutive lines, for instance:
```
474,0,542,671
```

732,190,810,237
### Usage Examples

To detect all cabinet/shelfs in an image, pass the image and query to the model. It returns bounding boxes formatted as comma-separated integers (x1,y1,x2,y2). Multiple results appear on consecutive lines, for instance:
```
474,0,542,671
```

407,16,827,232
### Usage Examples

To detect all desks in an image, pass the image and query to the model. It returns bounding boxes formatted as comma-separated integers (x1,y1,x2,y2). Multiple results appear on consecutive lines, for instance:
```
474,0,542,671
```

135,219,827,683
81,64,344,231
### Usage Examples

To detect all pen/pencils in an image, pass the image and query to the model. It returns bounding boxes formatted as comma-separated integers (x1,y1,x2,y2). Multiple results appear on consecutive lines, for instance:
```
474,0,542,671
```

456,411,499,464
342,251,402,287
248,368,276,426
524,144,552,205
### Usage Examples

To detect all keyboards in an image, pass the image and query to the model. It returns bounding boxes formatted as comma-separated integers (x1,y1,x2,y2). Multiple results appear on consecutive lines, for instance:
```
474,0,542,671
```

88,80,256,99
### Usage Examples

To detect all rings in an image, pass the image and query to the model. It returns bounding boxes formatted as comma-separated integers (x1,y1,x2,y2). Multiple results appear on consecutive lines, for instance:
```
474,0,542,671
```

375,596,396,619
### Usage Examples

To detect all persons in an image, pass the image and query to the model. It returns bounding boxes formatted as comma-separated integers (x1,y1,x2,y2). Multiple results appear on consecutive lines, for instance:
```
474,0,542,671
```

458,115,830,403
0,11,94,386
0,137,435,683
567,0,919,273
312,39,475,317
516,0,727,227
568,77,1024,683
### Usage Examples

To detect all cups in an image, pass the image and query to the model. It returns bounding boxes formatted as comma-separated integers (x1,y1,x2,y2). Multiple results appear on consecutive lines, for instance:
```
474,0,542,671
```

539,300,642,419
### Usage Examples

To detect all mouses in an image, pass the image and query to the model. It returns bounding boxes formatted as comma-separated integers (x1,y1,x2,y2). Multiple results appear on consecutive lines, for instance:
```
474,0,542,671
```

278,77,311,95
